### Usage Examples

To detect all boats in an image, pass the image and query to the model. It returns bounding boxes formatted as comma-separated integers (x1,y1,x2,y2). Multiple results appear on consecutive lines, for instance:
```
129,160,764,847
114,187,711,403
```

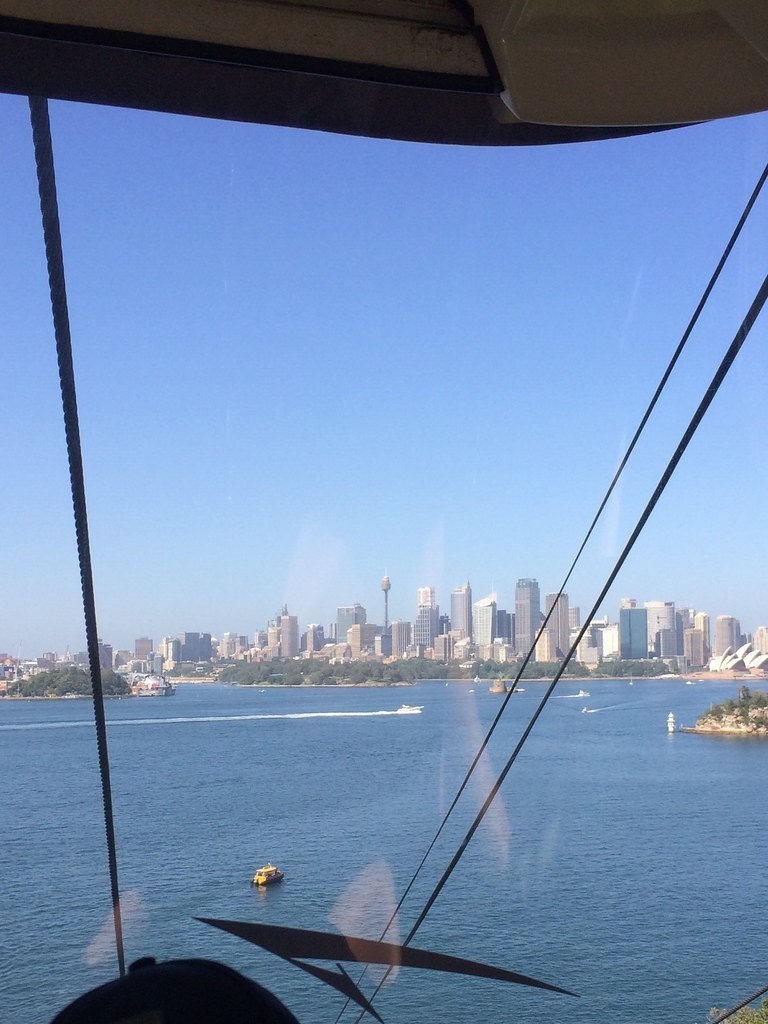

578,690,590,696
507,688,525,693
252,862,285,886
395,704,425,715
